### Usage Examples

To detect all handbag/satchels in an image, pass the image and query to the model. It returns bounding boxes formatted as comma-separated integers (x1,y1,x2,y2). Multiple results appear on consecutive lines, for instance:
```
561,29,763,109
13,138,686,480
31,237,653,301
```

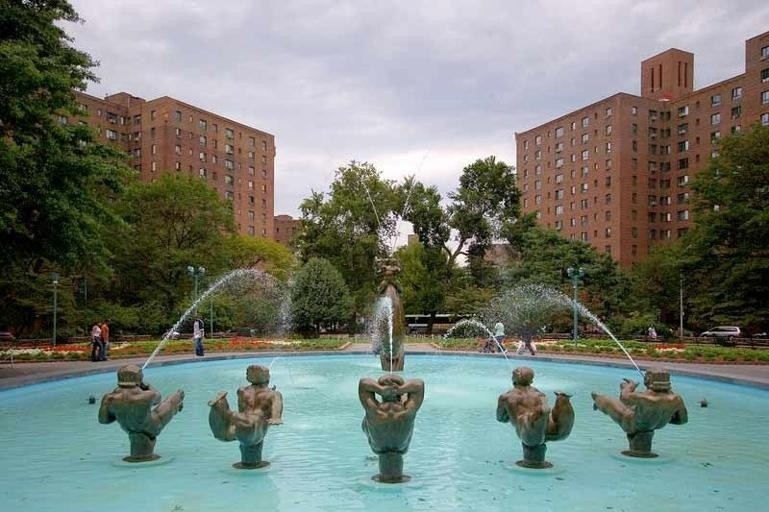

530,338,538,354
515,340,526,354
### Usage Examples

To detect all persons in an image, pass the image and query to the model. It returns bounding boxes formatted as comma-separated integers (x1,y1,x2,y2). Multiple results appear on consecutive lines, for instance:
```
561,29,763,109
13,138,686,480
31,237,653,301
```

91,319,106,361
496,366,574,447
101,319,110,355
358,376,425,455
518,319,535,356
207,364,285,446
494,320,507,354
193,310,204,356
591,368,687,435
98,365,185,437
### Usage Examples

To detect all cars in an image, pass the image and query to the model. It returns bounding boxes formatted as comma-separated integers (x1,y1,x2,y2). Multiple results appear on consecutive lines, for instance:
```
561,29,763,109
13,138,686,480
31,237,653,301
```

753,332,768,339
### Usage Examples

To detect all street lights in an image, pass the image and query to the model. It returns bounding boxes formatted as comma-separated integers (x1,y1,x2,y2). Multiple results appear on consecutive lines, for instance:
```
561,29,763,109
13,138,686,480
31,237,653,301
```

53,280,58,346
567,267,584,346
188,265,204,321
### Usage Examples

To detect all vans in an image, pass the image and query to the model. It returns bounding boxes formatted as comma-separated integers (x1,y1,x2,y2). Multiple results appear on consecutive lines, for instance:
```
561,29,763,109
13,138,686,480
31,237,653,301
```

700,326,742,341
0,332,16,341
